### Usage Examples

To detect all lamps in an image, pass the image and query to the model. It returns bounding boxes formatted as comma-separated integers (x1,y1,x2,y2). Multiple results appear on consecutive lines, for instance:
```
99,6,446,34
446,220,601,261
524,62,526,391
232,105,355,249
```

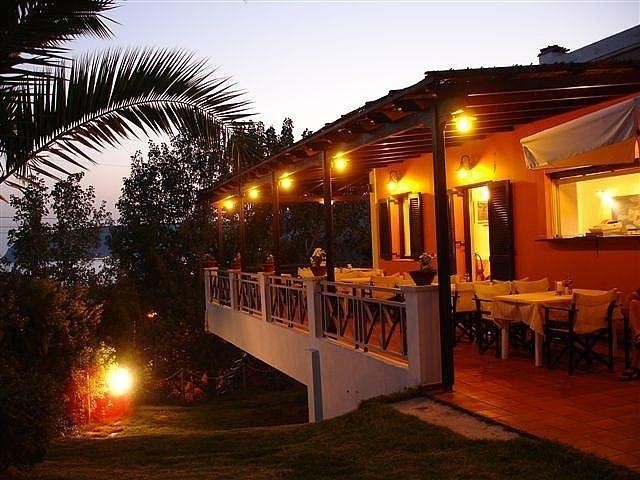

450,111,478,132
385,166,403,191
452,152,474,180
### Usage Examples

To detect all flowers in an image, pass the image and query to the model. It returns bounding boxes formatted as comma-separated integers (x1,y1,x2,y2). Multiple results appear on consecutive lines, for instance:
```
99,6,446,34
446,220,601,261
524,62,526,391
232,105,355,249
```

265,253,275,264
308,246,328,266
416,249,437,270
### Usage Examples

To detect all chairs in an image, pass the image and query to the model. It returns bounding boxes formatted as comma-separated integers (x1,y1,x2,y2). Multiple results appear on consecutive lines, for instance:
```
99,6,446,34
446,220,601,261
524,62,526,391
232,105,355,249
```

295,264,484,347
624,290,639,383
512,279,550,351
472,281,515,355
543,288,618,375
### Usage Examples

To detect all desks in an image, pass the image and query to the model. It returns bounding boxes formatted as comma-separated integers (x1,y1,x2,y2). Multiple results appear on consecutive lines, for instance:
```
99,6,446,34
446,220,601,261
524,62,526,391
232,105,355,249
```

493,289,571,368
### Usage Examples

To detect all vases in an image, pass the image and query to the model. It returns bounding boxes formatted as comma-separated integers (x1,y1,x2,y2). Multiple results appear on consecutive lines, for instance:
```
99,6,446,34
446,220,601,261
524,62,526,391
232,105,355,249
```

262,263,275,272
